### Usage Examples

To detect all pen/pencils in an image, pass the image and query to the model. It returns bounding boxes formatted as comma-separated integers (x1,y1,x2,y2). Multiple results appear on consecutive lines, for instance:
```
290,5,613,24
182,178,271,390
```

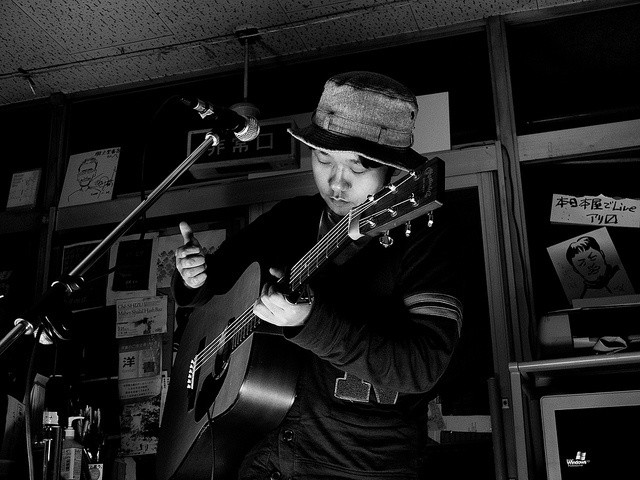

76,405,102,442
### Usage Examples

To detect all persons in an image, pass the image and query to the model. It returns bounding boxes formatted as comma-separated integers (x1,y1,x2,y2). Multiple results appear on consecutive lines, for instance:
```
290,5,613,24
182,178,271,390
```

170,69,463,480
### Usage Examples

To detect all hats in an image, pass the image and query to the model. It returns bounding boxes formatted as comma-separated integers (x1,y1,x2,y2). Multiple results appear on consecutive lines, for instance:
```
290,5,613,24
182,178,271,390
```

287,71,429,173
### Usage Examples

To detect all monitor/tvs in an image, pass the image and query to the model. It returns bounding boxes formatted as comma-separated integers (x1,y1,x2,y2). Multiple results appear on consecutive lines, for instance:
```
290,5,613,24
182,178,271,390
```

539,390,640,480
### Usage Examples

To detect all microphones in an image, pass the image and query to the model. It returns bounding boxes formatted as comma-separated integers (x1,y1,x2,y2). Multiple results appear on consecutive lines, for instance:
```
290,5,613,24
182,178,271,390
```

181,95,259,142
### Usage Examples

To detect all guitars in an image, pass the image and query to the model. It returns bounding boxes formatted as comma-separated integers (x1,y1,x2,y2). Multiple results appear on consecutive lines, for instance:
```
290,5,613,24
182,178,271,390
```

157,157,447,480
